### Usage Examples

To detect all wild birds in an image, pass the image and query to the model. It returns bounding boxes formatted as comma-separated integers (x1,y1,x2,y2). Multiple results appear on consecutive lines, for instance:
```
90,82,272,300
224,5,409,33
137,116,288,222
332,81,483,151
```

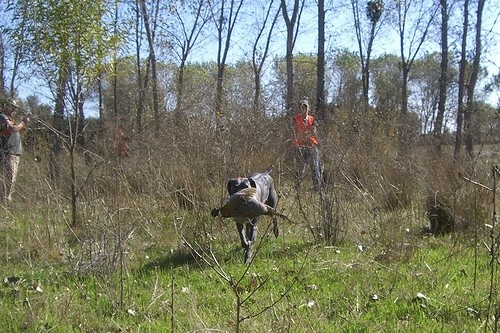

211,187,293,238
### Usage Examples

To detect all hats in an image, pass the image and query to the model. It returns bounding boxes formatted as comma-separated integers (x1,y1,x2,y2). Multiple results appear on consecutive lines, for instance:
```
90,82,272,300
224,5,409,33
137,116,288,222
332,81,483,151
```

299,100,309,107
7,99,20,107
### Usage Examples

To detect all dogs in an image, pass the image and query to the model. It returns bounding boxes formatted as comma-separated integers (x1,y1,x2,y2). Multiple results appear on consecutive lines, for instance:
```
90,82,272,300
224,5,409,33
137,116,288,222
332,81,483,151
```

228,164,281,266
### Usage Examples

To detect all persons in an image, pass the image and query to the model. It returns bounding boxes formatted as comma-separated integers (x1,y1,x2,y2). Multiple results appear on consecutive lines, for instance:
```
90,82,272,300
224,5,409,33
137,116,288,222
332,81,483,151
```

294,99,326,195
0,98,29,210
